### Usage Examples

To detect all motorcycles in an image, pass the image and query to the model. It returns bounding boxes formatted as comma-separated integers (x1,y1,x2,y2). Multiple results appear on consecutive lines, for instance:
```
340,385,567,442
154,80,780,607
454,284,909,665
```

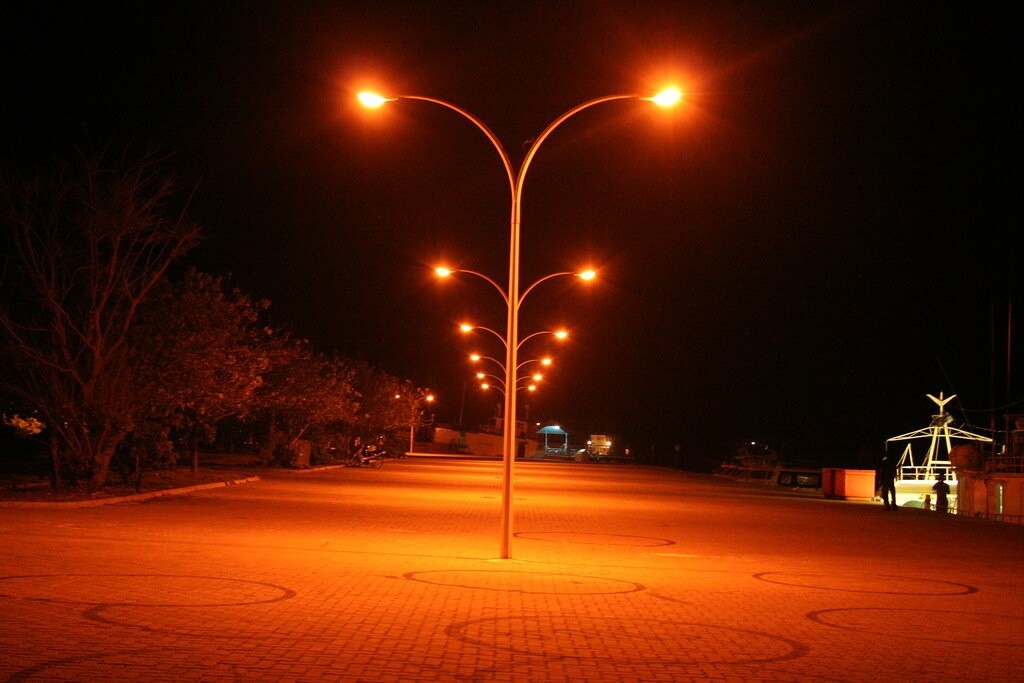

345,442,386,469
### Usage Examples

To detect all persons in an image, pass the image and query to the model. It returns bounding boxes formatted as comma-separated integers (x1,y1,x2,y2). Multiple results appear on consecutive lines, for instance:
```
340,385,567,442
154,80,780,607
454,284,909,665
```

876,451,898,512
932,474,950,512
354,435,377,467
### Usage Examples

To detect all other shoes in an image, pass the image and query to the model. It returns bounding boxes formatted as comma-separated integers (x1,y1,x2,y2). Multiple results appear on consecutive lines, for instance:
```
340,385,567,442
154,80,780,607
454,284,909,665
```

885,503,890,511
891,502,897,511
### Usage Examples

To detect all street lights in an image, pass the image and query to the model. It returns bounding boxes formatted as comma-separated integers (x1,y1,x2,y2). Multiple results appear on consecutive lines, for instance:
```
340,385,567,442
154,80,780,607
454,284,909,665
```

459,323,569,561
481,383,538,561
355,85,685,562
468,354,553,561
436,266,597,560
395,394,433,452
475,373,544,560
